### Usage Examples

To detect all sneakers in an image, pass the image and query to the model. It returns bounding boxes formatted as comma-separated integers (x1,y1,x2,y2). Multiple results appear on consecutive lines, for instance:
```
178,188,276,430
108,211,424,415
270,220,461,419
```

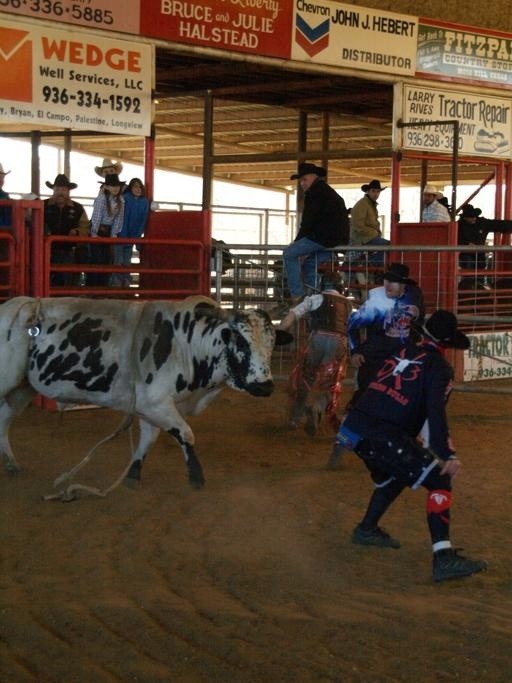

305,403,320,436
432,547,487,581
351,525,401,548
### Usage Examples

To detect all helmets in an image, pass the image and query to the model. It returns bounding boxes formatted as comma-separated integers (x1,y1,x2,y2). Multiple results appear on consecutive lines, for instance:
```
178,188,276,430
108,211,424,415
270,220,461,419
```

321,271,344,291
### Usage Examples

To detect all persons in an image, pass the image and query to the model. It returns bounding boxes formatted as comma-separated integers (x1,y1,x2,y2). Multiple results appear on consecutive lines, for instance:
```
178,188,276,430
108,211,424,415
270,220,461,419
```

275,271,353,436
325,263,430,472
458,203,512,289
282,163,350,304
351,179,389,266
421,185,451,222
0,157,149,299
336,311,488,582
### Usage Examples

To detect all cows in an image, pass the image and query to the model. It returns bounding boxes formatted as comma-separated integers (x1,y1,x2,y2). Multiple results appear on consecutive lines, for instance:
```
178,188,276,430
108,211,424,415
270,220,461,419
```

0,294,294,491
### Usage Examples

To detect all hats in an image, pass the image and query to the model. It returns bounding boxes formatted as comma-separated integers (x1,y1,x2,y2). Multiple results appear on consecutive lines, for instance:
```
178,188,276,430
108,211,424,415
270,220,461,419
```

95,158,122,177
45,174,77,189
97,173,125,186
376,262,417,285
362,179,387,192
460,204,482,217
291,163,327,180
0,162,10,174
421,185,444,200
410,310,471,349
